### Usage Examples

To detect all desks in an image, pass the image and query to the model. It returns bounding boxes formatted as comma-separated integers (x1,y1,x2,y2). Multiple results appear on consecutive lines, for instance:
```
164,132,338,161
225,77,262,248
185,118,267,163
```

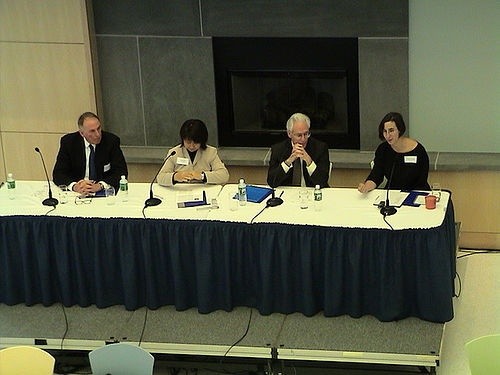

0,181,456,323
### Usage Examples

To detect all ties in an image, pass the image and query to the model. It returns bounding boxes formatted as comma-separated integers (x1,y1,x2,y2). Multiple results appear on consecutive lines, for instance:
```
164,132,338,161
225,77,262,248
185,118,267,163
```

292,158,301,188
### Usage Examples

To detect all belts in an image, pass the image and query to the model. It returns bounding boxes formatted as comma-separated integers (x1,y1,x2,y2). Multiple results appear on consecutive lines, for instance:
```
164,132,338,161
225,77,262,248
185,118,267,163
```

89,145,96,180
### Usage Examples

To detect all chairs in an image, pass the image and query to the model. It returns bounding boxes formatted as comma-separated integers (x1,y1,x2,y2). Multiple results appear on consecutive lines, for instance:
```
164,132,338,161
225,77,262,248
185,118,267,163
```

88,342,154,375
465,334,500,375
0,346,55,375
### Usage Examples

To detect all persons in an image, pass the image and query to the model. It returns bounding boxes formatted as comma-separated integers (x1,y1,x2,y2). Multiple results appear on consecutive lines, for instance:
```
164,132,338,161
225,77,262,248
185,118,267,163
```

157,119,228,186
53,112,128,193
266,113,330,188
358,112,431,193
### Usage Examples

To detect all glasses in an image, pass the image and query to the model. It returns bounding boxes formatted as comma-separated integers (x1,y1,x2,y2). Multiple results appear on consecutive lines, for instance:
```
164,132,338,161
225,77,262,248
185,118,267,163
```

75,195,93,205
292,131,308,139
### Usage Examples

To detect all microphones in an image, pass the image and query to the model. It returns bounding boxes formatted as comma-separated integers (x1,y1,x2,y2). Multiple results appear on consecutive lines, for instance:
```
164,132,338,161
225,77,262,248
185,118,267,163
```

145,151,176,206
380,159,398,215
266,155,285,206
34,148,59,206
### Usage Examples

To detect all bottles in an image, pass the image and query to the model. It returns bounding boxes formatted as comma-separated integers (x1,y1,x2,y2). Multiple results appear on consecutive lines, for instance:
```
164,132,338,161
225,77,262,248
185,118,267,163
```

238,179,247,205
7,173,16,199
119,175,128,202
313,184,323,208
231,195,240,211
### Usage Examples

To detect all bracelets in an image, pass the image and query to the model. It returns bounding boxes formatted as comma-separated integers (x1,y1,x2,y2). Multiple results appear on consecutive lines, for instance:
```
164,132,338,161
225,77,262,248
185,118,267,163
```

100,183,103,189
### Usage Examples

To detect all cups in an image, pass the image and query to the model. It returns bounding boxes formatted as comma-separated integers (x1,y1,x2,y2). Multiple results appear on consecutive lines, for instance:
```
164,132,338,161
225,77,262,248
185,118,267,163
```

425,196,436,209
211,193,218,209
105,186,115,204
430,183,442,202
58,185,67,204
299,188,308,209
